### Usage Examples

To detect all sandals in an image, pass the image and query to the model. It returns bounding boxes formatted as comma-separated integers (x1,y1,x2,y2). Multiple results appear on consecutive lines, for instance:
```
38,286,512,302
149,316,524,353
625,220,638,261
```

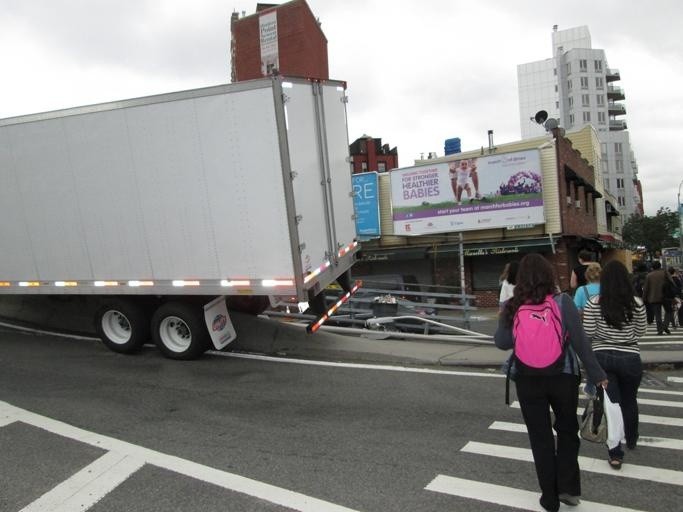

606,452,623,470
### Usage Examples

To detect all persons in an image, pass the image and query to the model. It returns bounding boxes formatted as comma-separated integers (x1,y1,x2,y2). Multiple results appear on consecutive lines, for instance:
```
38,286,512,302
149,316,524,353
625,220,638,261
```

498,260,520,313
625,258,683,338
580,260,647,471
498,179,541,195
569,250,594,290
496,263,511,299
449,159,477,206
571,262,602,312
492,252,609,511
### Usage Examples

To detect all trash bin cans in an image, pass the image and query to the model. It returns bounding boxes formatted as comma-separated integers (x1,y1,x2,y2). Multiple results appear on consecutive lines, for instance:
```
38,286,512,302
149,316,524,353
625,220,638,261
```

370,303,397,333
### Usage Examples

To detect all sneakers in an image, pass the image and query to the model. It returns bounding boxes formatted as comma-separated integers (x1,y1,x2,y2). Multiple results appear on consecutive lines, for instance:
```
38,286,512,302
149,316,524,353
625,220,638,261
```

646,319,683,336
559,492,582,507
540,494,560,512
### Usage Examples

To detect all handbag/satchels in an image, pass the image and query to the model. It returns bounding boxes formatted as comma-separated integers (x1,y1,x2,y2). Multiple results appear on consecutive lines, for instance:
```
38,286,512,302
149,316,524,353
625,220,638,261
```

579,383,608,444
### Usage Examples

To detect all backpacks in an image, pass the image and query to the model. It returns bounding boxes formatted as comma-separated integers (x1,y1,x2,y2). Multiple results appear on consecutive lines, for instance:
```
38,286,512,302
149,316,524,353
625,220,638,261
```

511,290,567,371
